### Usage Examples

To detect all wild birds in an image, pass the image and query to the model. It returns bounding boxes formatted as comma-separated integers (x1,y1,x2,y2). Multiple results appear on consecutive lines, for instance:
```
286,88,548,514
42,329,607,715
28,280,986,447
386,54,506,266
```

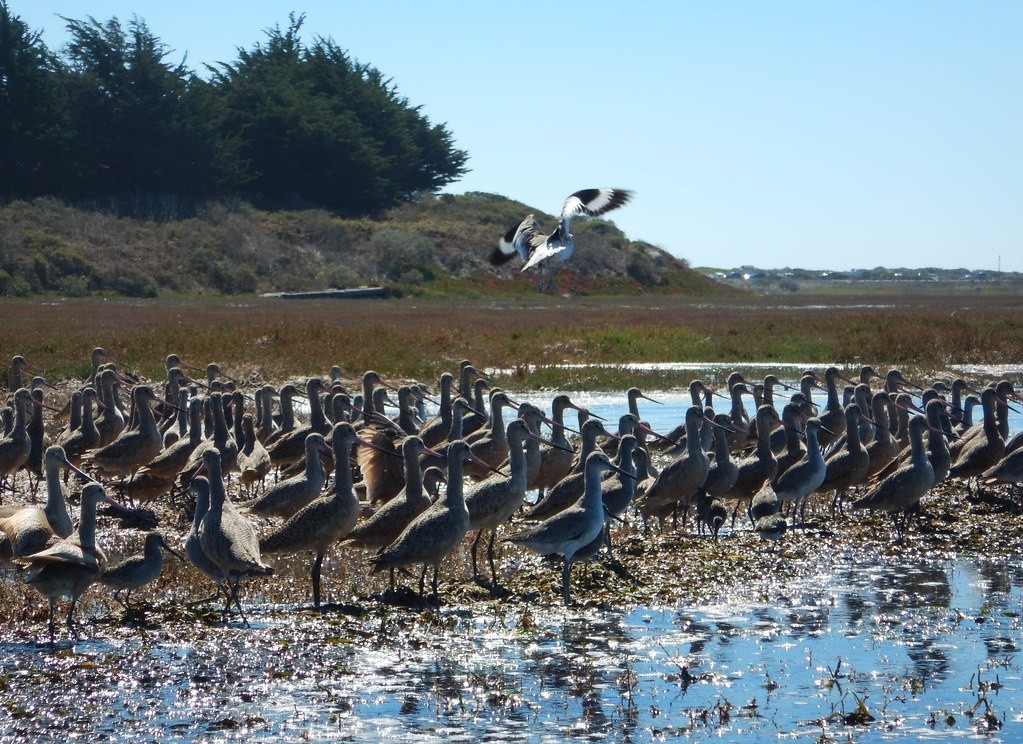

0,347,1022,631
489,188,637,293
